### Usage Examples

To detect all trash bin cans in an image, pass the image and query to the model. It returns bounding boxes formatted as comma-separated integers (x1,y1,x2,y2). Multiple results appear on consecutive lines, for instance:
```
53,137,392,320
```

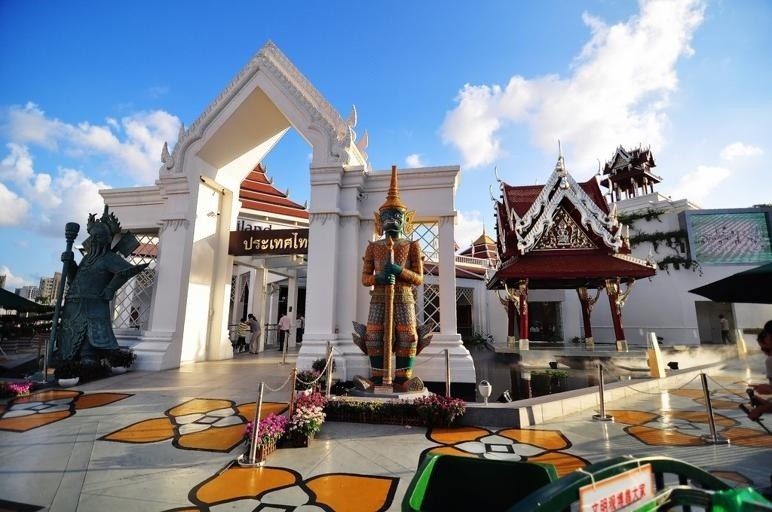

401,454,772,512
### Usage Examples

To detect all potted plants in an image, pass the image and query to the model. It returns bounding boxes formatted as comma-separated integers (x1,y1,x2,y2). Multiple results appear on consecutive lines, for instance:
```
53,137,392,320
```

110,346,136,374
57,367,79,387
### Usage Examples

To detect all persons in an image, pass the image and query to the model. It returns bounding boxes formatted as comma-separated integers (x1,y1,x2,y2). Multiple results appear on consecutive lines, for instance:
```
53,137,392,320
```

246,314,261,354
362,198,423,392
296,315,303,342
300,313,304,334
277,311,290,351
748,321,772,421
234,318,248,353
51,223,139,382
718,314,731,344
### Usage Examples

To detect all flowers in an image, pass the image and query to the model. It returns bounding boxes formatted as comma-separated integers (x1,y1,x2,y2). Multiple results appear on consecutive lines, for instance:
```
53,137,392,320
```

285,357,415,440
412,395,467,424
245,413,289,446
